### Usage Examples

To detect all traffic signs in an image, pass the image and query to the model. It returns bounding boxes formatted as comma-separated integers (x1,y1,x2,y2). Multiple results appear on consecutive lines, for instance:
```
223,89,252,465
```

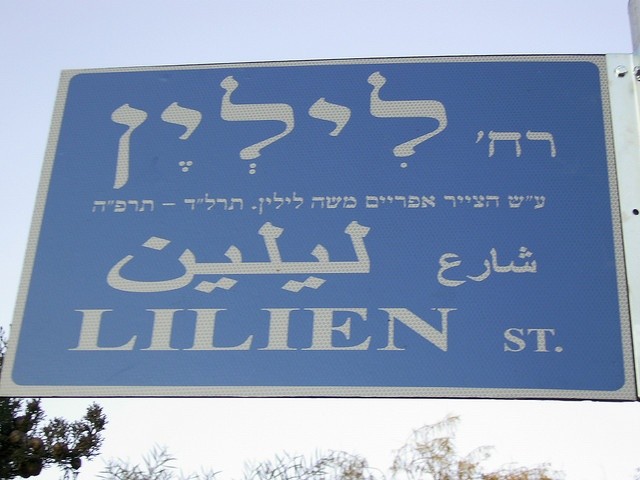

1,52,640,404
